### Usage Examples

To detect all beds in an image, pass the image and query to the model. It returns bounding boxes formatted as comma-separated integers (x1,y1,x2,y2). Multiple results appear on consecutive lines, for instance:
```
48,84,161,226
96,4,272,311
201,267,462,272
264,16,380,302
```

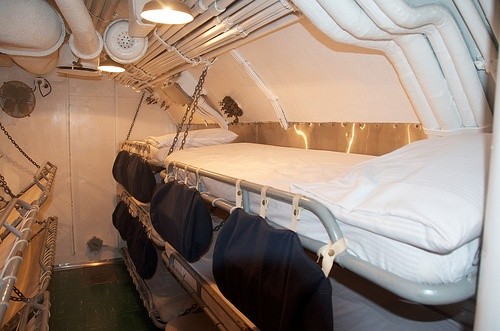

112,129,493,331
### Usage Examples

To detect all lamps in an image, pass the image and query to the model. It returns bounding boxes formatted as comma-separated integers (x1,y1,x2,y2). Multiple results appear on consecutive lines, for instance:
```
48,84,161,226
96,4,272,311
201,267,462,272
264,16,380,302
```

97,57,125,73
139,0,195,25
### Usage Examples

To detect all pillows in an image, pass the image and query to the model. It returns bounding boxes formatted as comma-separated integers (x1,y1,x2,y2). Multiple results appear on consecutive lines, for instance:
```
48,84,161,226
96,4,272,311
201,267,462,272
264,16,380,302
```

290,132,492,254
144,128,238,149
